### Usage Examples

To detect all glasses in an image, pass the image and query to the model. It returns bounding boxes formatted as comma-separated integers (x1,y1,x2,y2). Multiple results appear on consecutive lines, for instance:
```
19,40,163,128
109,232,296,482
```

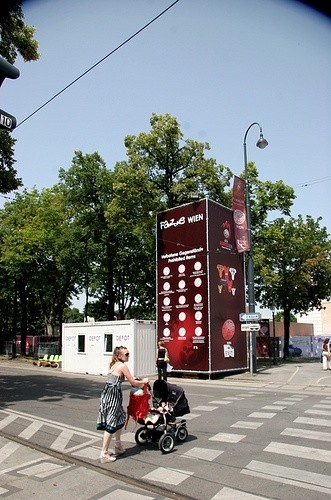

121,353,129,356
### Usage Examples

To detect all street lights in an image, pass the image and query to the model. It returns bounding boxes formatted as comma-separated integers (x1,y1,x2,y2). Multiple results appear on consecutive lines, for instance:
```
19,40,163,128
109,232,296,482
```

242,122,267,377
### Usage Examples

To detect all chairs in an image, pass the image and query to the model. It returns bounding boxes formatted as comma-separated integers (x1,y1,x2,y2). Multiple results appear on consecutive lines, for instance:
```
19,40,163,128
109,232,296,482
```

38,354,63,369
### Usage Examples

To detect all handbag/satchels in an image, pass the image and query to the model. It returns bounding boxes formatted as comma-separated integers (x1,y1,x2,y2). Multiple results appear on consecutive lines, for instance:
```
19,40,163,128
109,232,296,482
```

164,349,170,362
127,382,152,421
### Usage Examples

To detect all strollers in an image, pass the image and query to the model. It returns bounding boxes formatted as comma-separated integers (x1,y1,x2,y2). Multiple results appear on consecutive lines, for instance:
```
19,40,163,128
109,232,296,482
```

129,377,189,454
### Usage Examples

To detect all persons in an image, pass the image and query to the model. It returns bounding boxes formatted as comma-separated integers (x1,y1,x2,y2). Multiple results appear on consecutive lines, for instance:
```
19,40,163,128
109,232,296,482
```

322,338,331,370
96,346,150,464
155,341,169,383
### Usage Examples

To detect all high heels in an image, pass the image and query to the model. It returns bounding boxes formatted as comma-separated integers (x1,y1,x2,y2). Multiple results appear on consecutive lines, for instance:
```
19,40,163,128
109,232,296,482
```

114,441,126,454
100,451,117,464
